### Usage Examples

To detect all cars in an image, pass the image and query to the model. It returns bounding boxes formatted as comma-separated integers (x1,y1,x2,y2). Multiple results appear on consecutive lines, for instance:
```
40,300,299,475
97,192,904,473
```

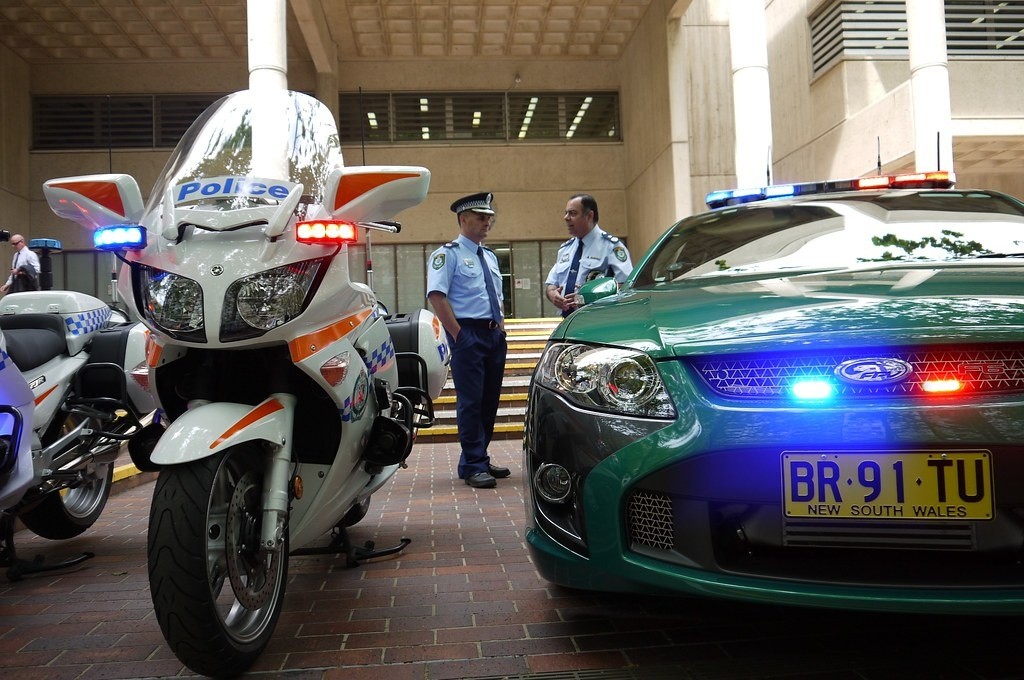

525,172,1024,617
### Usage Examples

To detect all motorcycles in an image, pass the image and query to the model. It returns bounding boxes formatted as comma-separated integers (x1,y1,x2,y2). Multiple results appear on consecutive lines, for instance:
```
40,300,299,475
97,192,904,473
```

0,230,143,583
40,89,436,680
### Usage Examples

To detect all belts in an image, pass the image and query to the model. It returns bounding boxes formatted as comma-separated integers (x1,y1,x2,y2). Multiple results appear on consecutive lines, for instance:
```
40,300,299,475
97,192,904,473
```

459,318,499,329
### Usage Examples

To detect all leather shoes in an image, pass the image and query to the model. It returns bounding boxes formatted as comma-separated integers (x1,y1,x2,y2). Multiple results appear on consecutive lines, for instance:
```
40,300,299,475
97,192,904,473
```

465,473,497,488
489,463,510,478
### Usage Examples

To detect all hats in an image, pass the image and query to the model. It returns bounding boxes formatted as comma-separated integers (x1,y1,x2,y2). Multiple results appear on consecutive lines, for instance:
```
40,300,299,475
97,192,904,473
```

585,267,613,282
450,192,495,216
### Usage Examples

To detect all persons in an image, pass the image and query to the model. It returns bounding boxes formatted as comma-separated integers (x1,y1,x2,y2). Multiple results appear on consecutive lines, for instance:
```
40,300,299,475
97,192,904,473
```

425,190,510,487
0,233,42,295
545,193,633,319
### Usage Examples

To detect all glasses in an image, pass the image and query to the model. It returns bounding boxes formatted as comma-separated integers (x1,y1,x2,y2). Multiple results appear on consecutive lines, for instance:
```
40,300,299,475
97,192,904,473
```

11,240,22,245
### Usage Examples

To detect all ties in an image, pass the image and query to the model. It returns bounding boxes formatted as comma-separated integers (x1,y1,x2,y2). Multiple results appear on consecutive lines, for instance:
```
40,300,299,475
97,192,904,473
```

477,246,501,325
562,240,584,318
12,253,19,281
